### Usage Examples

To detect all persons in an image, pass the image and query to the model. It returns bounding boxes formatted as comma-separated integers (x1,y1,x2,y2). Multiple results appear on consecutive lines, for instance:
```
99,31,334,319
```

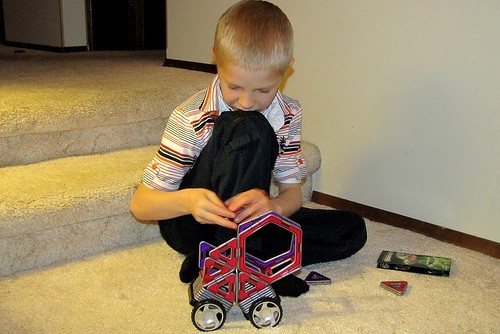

129,0,367,297
